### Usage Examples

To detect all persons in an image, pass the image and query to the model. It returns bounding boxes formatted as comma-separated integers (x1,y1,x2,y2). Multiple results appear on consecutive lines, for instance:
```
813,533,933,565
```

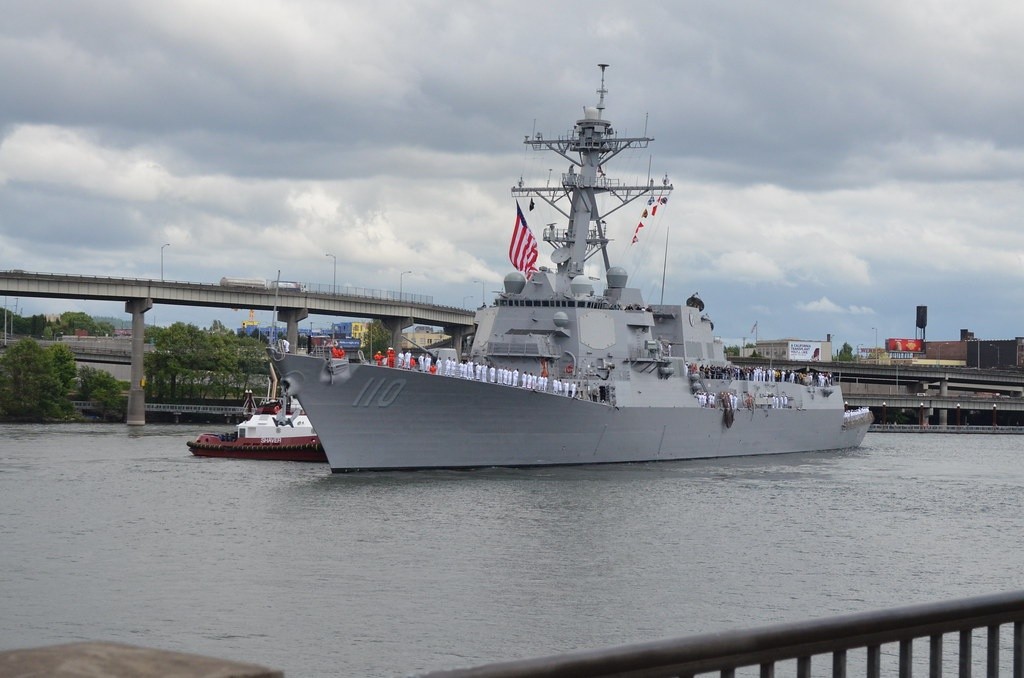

553,378,606,402
459,361,519,386
375,351,386,366
387,347,396,368
625,303,652,311
332,346,344,358
522,371,547,391
283,338,290,353
684,364,834,386
695,391,788,410
845,406,869,422
398,350,456,377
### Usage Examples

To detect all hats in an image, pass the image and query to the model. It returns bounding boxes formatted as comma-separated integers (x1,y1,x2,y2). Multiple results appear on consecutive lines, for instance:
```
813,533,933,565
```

378,351,381,354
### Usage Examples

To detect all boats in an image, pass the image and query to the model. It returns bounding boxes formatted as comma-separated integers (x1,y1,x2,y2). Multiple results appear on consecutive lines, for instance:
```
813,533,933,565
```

266,63,875,473
186,396,326,462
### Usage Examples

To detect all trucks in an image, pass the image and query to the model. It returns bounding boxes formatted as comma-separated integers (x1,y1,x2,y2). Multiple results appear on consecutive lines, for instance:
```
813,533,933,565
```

270,281,309,293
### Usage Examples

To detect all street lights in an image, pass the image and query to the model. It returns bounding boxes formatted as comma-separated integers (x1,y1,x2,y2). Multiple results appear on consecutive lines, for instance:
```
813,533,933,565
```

990,344,999,364
474,281,484,302
883,402,886,425
161,244,170,282
939,343,949,368
920,403,924,425
872,328,878,364
957,403,961,425
845,401,848,412
993,404,996,426
400,271,411,300
326,254,336,294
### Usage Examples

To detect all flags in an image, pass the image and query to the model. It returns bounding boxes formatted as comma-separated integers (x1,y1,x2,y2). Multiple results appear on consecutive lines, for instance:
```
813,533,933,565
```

632,197,660,245
509,198,538,277
751,322,757,332
